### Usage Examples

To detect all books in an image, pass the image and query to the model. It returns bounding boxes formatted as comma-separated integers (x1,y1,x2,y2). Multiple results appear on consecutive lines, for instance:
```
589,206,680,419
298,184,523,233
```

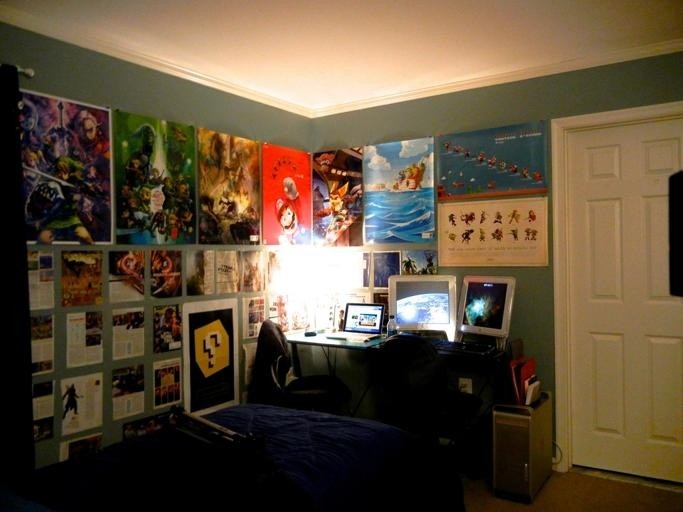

509,354,543,406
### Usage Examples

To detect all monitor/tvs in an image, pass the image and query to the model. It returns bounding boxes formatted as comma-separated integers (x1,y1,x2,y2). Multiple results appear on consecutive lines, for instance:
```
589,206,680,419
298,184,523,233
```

459,274,516,338
387,273,456,336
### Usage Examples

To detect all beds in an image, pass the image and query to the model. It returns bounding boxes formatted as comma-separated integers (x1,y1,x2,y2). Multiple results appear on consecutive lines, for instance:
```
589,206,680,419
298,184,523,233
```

0,402,467,510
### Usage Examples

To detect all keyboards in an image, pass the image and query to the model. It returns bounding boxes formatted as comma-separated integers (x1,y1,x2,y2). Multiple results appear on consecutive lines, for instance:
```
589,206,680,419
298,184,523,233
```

433,340,494,357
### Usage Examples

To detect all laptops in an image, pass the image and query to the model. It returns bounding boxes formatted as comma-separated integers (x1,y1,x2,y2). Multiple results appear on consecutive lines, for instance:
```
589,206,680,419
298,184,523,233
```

326,302,385,343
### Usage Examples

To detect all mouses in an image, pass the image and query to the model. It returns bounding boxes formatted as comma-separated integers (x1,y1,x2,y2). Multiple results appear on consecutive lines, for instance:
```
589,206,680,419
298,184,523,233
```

357,336,370,342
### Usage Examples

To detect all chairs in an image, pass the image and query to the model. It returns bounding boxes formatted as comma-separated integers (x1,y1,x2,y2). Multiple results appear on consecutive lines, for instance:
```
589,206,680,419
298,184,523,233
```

258,319,350,417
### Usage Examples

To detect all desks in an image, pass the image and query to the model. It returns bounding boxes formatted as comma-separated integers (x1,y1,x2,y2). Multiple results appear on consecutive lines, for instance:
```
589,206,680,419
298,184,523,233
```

279,326,512,482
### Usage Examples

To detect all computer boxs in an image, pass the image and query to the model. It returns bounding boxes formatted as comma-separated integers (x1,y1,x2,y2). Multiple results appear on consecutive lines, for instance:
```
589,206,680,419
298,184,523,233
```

493,390,553,502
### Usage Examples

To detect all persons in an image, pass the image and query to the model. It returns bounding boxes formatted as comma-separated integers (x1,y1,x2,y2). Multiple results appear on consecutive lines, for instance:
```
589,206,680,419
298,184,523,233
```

83,309,145,348
155,365,181,406
336,309,345,330
151,307,182,353
